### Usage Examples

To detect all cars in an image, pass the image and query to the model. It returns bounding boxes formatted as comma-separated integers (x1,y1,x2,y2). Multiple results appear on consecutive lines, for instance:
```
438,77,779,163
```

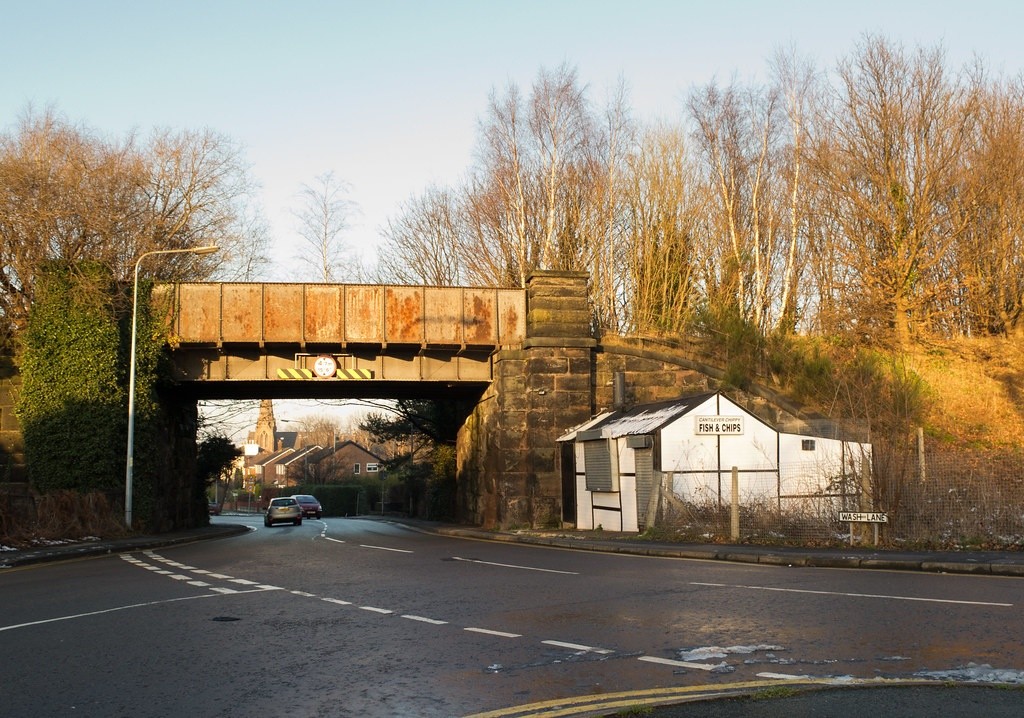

208,501,221,514
290,495,322,519
264,497,302,527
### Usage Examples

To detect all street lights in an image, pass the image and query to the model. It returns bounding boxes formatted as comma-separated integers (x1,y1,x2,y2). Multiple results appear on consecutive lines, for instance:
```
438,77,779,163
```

125,246,221,529
281,419,308,484
